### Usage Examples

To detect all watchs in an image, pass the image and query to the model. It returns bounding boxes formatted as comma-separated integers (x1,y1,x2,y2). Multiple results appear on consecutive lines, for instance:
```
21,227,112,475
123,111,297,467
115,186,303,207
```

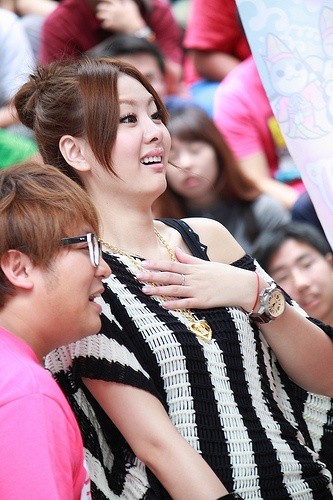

249,281,285,324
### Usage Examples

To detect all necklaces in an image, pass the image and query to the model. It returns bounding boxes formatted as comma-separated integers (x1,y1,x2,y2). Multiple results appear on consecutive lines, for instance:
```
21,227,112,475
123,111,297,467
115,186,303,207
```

97,223,213,340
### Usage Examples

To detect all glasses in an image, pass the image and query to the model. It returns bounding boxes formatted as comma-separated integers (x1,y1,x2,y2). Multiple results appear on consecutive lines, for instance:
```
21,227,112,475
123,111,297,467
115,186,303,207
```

15,233,102,267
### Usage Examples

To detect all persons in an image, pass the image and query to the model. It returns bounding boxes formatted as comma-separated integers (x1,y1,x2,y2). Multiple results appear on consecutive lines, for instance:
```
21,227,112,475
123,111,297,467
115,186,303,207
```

14,58,333,500
0,0,333,500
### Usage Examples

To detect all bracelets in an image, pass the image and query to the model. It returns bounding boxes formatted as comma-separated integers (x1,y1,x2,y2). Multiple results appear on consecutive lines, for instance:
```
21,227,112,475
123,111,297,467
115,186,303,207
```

245,271,259,317
